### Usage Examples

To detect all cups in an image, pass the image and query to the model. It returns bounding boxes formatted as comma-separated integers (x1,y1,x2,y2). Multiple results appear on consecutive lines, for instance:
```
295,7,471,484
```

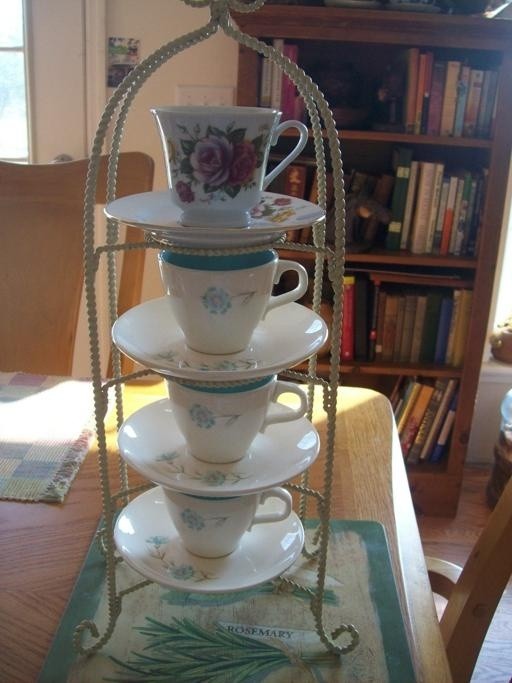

152,106,307,227
159,247,309,353
160,485,294,561
167,380,307,467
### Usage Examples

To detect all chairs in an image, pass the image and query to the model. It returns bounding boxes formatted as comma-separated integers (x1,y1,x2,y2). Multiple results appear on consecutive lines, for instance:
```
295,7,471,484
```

2,154,157,384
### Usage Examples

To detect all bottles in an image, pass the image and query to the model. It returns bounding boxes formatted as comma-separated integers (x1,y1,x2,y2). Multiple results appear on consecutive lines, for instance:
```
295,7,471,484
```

489,324,511,365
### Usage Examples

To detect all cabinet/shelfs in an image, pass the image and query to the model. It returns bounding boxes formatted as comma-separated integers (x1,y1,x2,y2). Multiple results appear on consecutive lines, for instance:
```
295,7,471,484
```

232,0,512,524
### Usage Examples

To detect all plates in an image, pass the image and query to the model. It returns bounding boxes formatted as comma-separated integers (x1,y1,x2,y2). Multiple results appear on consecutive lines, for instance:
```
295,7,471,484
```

102,186,323,244
120,399,323,498
115,486,308,595
110,294,331,382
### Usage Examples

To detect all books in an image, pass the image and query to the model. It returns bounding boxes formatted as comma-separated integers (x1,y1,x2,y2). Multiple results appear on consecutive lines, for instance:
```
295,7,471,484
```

268,143,490,256
388,373,461,467
401,47,498,138
261,40,307,124
272,261,472,368
